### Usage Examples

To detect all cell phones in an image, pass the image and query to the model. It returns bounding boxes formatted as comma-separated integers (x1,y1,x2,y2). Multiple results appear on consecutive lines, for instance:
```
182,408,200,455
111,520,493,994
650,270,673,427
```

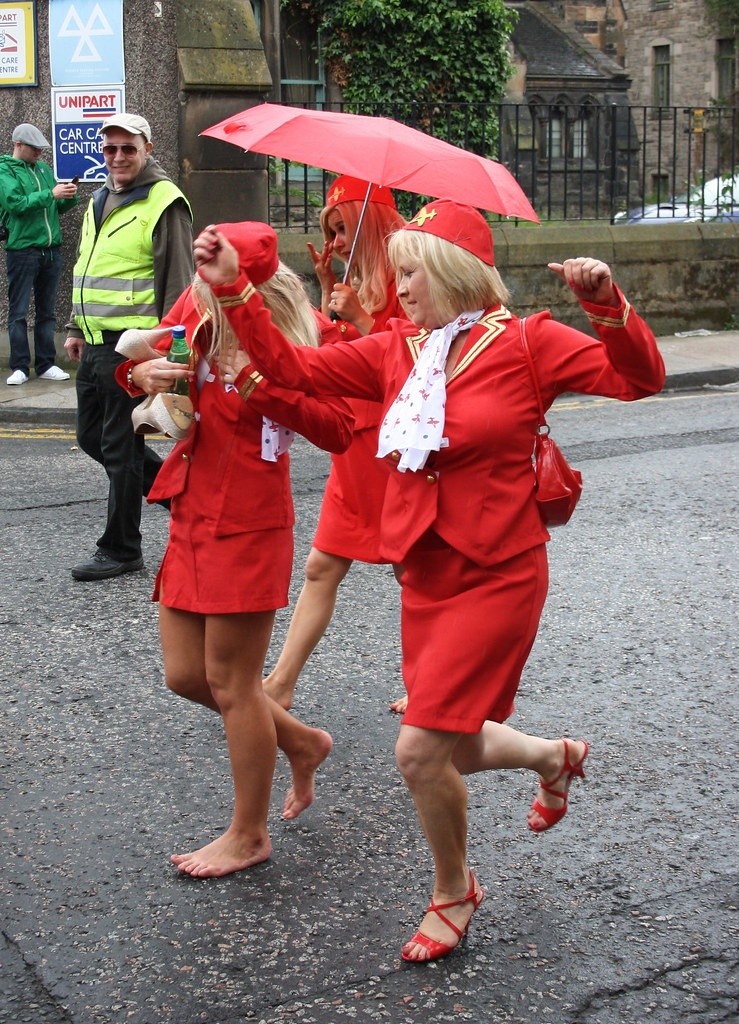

71,174,79,184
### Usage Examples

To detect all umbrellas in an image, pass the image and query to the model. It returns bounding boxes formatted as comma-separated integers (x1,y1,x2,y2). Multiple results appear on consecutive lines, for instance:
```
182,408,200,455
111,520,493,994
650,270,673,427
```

201,102,540,284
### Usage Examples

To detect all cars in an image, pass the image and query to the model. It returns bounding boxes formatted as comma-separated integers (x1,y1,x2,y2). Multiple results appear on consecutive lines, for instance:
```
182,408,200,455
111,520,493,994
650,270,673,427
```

615,173,739,222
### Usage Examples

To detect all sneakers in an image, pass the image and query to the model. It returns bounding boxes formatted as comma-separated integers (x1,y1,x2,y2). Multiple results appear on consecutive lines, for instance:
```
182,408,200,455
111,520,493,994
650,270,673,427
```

6,363,71,386
72,544,144,579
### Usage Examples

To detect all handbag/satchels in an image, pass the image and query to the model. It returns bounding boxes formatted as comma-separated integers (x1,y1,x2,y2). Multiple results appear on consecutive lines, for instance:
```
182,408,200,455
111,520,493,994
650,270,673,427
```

532,428,584,528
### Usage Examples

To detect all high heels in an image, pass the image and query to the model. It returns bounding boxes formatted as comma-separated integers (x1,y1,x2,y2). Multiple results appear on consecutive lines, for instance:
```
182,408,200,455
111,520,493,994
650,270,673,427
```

115,327,192,439
401,869,486,964
528,736,589,835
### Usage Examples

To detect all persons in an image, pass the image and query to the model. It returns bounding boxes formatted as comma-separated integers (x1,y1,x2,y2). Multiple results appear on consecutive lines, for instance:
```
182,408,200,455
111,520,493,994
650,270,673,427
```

0,124,80,385
114,220,334,879
65,112,195,581
192,175,666,962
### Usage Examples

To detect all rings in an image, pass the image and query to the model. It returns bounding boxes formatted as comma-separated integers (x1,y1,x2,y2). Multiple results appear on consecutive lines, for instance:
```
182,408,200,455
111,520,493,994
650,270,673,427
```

331,299,336,305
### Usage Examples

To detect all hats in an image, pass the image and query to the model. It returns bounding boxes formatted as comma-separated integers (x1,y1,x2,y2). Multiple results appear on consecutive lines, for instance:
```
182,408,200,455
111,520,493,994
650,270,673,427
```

403,199,499,269
12,123,52,149
201,221,280,286
96,112,154,143
324,174,396,211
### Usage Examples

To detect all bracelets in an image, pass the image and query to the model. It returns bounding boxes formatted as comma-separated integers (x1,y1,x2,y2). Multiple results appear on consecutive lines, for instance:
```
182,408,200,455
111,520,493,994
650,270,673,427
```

127,365,137,391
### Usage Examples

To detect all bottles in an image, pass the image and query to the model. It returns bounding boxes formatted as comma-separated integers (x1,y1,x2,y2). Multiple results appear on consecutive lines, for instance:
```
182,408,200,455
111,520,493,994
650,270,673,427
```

167,325,192,397
